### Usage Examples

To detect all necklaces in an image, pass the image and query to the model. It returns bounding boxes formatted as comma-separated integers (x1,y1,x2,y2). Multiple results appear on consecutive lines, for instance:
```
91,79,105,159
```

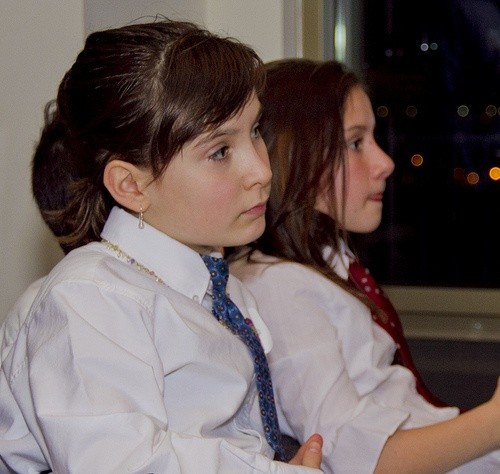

99,234,229,328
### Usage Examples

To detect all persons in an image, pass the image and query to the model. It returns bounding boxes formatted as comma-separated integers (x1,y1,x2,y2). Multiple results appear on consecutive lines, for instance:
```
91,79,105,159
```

234,56,500,472
0,10,324,474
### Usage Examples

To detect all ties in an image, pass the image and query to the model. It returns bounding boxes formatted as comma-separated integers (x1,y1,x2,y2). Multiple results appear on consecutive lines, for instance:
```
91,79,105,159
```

202,255,301,463
349,258,448,408
